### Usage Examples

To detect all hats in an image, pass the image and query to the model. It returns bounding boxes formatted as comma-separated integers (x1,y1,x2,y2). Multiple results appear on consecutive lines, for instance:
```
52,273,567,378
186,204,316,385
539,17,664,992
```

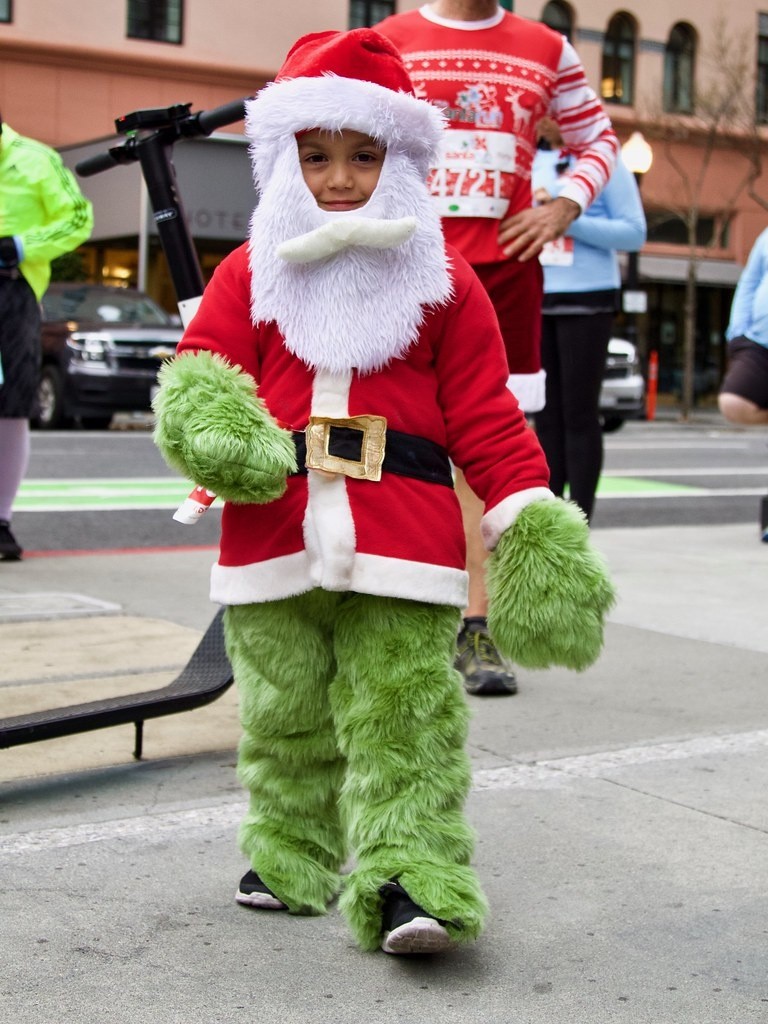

244,28,451,376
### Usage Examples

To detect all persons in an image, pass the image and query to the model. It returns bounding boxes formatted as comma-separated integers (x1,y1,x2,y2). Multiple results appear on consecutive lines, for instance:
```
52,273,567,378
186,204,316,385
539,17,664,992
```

717,221,768,426
0,112,94,560
154,28,617,955
363,0,647,696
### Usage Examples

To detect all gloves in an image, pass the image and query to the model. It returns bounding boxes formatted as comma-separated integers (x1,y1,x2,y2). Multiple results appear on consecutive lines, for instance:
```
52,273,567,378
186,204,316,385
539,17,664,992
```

0,236,23,270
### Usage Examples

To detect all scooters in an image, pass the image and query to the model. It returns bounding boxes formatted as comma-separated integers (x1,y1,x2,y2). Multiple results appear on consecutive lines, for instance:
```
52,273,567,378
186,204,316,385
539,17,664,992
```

0,96,255,756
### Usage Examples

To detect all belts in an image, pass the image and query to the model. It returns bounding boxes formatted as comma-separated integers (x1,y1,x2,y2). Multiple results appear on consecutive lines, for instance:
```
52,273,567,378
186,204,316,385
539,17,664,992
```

284,414,455,488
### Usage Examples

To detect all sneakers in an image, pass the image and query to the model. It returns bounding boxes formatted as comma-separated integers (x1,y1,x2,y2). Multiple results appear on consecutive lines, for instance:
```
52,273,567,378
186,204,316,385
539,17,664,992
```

378,878,449,954
450,621,518,695
235,869,283,909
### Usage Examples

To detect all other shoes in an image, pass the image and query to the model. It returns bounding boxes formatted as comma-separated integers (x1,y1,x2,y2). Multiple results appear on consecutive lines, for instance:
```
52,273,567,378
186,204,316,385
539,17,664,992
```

0,519,22,559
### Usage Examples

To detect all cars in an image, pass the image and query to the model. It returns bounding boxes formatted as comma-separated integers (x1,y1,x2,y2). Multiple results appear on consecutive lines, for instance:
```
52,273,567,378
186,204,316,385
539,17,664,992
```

29,277,187,429
511,336,646,432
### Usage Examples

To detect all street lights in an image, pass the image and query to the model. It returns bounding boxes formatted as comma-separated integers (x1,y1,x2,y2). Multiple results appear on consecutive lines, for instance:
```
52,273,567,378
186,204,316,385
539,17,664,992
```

621,130,653,334
